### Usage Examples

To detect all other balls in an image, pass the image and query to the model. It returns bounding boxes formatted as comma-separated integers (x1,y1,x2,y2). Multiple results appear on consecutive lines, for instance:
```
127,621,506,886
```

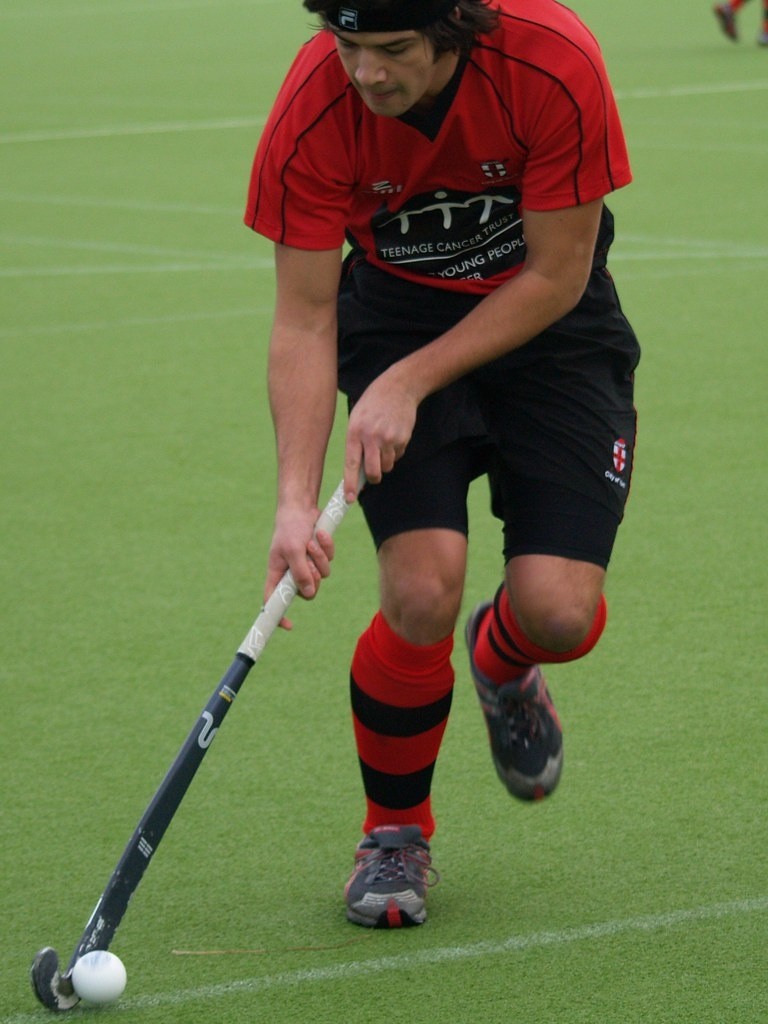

72,949,129,1005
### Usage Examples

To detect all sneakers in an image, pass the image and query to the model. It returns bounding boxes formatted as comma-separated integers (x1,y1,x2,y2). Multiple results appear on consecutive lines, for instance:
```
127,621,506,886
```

345,821,439,929
465,599,565,799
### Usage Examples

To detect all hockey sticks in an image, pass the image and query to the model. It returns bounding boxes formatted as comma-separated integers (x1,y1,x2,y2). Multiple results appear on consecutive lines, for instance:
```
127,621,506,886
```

26,459,373,1014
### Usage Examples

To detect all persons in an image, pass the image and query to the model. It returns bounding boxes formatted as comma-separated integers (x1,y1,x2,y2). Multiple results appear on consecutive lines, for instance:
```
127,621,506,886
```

712,0,768,48
242,0,641,928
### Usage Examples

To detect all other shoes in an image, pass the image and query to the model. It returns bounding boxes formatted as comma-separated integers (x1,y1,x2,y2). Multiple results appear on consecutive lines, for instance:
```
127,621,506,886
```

715,5,738,41
